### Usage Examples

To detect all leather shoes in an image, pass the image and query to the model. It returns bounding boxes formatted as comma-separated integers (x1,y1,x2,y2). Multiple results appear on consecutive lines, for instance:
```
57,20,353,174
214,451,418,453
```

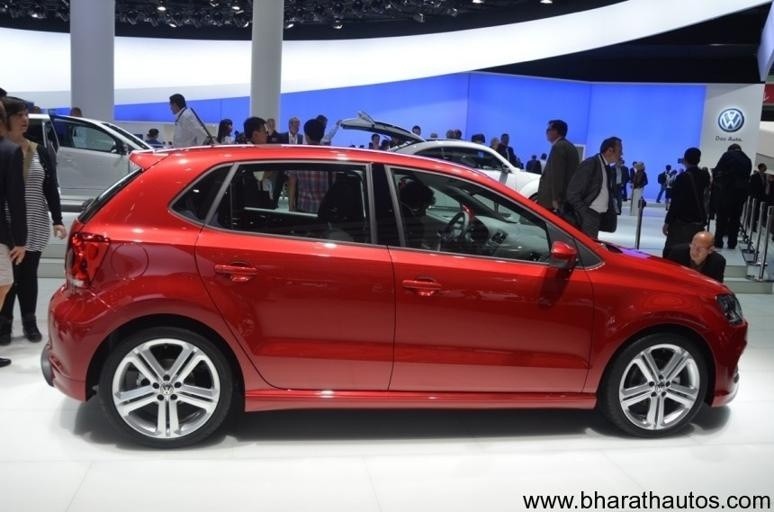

0,358,10,367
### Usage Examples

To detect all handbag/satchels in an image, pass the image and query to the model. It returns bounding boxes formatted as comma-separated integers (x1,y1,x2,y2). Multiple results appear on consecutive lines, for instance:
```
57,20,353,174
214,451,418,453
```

599,208,617,232
669,216,704,241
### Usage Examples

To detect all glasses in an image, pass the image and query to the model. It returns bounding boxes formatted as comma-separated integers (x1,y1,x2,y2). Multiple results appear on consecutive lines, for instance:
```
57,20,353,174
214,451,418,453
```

688,243,710,252
546,128,552,134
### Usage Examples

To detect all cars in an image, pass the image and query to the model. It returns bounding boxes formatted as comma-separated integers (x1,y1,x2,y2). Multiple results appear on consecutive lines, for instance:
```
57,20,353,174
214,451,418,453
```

336,111,542,205
41,144,748,447
26,113,157,200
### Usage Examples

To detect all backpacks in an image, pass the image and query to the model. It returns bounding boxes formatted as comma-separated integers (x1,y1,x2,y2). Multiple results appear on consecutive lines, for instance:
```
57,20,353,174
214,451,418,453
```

658,171,665,185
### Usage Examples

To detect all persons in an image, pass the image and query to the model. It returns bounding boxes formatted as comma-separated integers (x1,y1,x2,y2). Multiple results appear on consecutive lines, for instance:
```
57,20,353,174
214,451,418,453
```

630,162,638,187
412,125,421,135
317,114,342,144
380,140,389,150
472,134,485,145
536,120,579,215
662,147,707,267
669,231,727,284
656,165,671,202
526,155,542,174
145,128,163,147
713,143,752,249
244,116,273,200
266,118,281,143
747,163,768,232
490,137,500,150
455,130,462,140
70,107,82,116
0,98,67,348
169,94,208,145
566,137,624,241
621,161,630,201
387,180,435,248
372,133,380,149
539,153,548,174
281,117,303,144
212,118,240,145
447,129,454,138
284,119,337,214
630,161,648,216
32,106,40,112
665,169,677,210
498,134,519,167
0,100,28,368
611,158,623,215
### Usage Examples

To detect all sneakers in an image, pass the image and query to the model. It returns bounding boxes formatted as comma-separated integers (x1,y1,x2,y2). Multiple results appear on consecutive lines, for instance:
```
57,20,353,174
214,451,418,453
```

0,322,11,345
24,320,41,343
